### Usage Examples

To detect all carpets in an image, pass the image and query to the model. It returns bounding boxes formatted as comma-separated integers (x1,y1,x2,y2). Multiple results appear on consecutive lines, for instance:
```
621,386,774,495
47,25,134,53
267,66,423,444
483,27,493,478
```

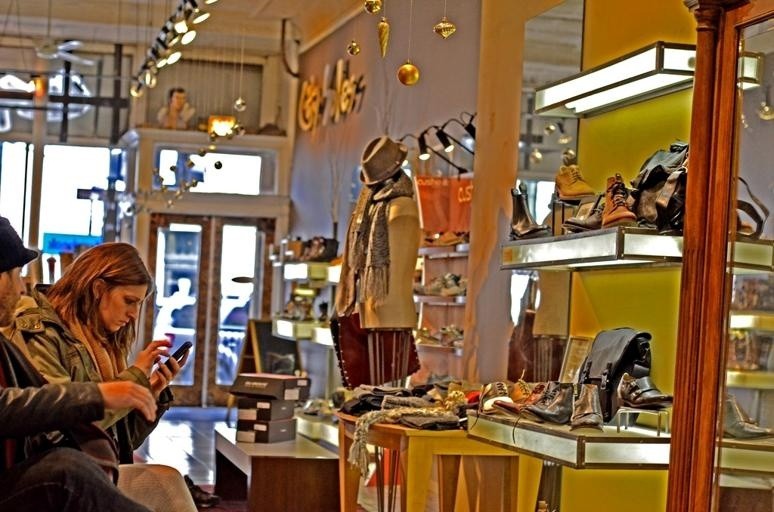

193,483,368,511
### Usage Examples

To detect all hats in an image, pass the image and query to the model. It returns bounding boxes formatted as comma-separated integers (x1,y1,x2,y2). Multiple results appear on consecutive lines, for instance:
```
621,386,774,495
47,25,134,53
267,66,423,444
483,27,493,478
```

358,133,408,185
0,216,41,275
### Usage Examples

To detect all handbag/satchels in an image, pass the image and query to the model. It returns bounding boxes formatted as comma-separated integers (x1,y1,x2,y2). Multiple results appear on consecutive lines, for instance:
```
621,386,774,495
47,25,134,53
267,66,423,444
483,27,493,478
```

326,309,424,393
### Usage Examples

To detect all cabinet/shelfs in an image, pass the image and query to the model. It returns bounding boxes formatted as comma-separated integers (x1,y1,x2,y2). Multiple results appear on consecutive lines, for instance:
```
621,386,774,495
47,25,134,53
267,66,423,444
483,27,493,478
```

465,38,698,475
725,307,773,393
313,263,347,350
412,246,468,353
713,47,773,478
272,260,331,343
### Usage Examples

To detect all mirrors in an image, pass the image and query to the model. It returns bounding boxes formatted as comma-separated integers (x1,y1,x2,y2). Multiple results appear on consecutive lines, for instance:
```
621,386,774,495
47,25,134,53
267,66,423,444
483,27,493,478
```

658,0,772,511
503,0,586,392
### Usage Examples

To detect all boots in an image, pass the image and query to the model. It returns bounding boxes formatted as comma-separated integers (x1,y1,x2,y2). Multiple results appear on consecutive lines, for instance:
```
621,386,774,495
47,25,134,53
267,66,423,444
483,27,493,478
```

602,174,636,229
476,375,607,430
715,393,774,440
551,161,595,199
275,236,468,351
503,188,552,240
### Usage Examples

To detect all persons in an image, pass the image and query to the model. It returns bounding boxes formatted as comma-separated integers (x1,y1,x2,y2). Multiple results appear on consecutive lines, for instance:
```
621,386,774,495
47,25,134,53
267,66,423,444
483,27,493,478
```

0,215,166,511
157,87,194,128
47,241,201,511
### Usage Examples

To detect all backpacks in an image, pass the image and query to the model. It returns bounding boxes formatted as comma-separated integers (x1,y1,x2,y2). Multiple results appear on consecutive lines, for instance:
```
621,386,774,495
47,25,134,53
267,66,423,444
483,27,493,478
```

633,139,688,232
575,324,655,409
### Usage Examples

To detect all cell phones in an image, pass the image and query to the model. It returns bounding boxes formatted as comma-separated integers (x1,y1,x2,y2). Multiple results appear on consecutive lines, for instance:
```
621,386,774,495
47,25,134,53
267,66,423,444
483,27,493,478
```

158,341,193,372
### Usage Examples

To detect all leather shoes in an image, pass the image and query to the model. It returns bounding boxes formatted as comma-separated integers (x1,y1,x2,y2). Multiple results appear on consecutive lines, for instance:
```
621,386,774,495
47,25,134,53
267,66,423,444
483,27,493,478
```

616,371,675,409
561,191,602,232
183,475,221,506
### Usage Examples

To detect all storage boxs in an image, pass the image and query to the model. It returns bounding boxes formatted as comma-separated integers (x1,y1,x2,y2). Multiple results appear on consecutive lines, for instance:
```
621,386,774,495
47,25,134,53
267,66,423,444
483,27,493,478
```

230,370,312,446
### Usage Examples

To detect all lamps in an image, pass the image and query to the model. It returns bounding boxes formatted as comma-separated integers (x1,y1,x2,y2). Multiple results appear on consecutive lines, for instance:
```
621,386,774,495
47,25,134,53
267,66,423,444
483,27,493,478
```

416,125,475,161
126,2,218,98
540,118,575,146
435,118,469,153
395,132,469,174
459,109,478,140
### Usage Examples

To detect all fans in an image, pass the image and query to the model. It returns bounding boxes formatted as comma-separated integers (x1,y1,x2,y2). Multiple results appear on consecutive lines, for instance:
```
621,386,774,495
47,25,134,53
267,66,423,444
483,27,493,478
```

27,1,94,69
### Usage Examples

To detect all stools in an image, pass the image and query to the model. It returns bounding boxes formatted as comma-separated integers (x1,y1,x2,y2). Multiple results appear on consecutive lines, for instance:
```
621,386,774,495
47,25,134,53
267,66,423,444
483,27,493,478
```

208,424,344,512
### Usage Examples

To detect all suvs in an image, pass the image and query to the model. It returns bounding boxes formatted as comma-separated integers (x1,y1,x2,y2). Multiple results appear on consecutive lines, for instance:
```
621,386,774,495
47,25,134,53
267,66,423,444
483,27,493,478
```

146,283,252,430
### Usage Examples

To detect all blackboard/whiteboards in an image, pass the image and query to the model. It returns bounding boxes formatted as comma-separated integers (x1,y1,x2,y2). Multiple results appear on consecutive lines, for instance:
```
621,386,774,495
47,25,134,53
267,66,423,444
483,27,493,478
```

248,320,303,373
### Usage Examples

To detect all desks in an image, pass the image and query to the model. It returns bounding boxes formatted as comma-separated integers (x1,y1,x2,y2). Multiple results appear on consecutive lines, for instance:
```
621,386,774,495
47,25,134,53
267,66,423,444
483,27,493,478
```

333,409,549,510
